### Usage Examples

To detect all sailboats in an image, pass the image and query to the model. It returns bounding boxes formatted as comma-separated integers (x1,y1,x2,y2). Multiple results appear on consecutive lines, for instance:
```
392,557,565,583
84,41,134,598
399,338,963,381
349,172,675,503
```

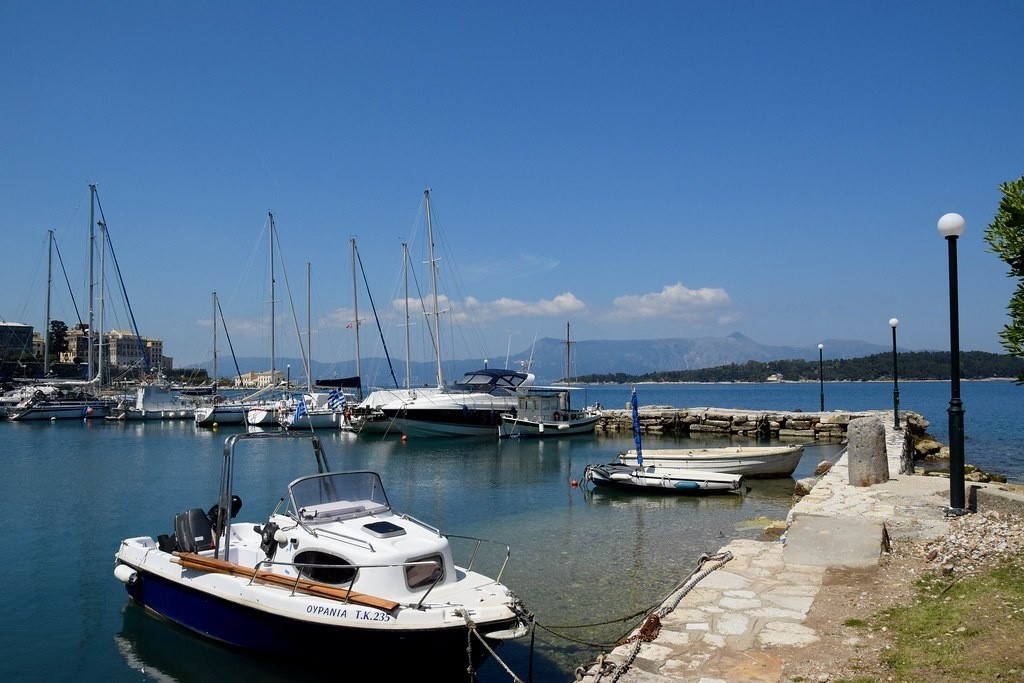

500,322,601,436
351,188,497,434
0,183,400,430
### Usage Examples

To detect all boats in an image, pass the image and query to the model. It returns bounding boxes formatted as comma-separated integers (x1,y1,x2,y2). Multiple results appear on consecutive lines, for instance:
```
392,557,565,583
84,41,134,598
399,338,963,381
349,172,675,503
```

382,332,566,441
618,445,805,479
113,398,530,683
583,381,744,495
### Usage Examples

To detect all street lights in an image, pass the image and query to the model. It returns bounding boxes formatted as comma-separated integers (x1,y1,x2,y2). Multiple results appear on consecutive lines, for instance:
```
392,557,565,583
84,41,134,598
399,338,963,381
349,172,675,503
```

818,344,824,411
937,212,965,510
890,318,902,431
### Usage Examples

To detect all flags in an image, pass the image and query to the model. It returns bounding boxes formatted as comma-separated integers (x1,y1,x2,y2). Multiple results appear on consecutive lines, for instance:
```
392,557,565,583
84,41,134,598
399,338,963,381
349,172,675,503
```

292,398,308,426
328,387,347,412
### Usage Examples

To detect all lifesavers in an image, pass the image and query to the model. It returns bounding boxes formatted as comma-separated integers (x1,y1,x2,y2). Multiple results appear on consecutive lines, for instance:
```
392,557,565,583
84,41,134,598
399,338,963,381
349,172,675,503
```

553,411,561,421
